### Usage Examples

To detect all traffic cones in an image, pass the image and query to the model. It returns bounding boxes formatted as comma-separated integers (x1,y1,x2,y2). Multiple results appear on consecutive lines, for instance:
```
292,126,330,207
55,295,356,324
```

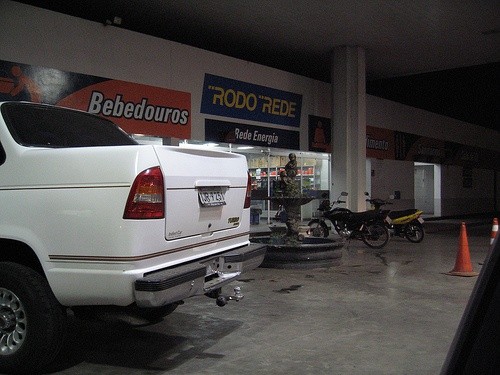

441,222,480,276
490,218,500,249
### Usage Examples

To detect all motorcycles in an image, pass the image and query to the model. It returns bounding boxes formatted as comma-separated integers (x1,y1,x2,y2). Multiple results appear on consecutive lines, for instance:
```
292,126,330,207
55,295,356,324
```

364,192,425,243
306,192,391,249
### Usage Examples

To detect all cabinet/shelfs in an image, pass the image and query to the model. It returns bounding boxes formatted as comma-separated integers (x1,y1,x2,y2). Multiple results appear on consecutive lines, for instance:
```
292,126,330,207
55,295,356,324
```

249,166,315,222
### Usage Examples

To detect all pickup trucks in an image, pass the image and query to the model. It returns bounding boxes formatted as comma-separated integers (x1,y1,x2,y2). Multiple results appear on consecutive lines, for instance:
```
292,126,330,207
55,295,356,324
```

0,100,266,375
